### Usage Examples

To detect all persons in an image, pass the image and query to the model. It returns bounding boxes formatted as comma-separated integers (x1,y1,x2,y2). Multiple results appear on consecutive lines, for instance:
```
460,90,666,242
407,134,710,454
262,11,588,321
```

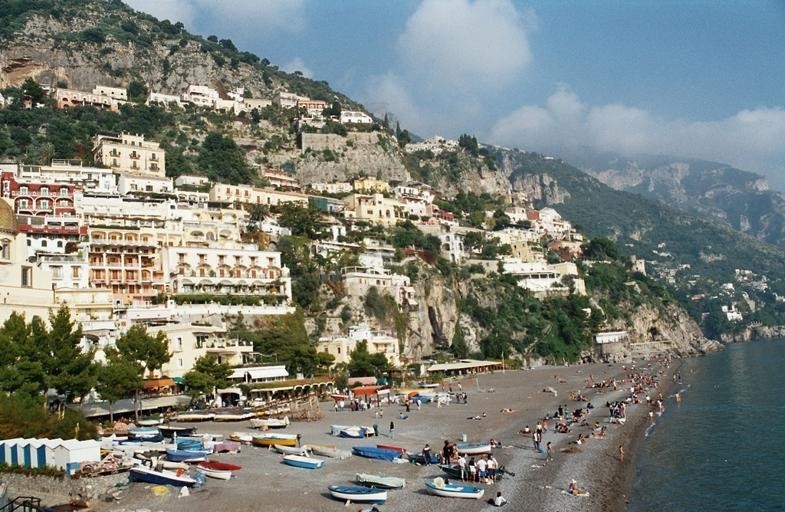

419,433,503,488
388,421,395,440
571,489,588,495
567,478,578,489
498,407,513,414
495,491,510,508
333,381,487,420
517,358,692,463
372,421,378,436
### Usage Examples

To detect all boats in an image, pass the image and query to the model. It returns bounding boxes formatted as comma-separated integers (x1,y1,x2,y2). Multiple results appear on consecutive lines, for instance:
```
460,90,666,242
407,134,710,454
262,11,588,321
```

331,381,449,407
